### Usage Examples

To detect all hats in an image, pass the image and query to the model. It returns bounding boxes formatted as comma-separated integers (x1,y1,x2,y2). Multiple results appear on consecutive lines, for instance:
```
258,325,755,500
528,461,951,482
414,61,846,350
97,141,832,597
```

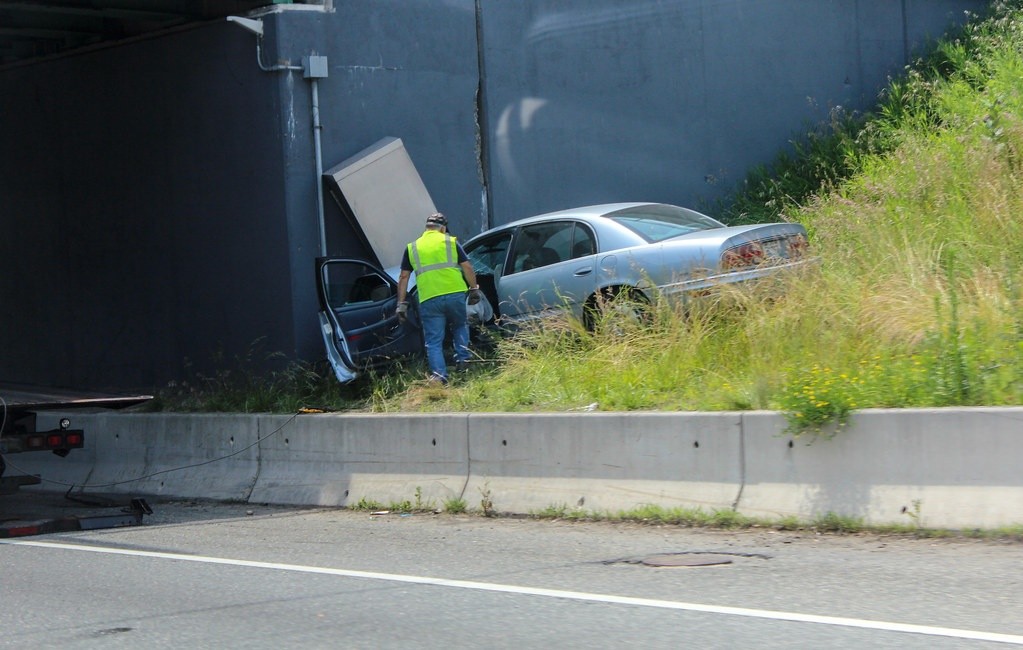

427,213,449,233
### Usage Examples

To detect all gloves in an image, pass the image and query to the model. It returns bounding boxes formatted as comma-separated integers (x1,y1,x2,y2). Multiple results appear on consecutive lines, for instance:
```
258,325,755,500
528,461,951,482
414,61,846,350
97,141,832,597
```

396,300,409,325
468,284,480,305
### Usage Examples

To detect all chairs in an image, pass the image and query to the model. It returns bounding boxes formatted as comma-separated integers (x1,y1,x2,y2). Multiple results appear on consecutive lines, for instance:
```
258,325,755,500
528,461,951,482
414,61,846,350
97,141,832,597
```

571,238,592,258
521,248,560,271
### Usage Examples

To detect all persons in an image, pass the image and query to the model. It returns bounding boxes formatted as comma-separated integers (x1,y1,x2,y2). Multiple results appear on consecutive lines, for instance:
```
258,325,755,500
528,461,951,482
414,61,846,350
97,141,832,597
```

395,213,482,386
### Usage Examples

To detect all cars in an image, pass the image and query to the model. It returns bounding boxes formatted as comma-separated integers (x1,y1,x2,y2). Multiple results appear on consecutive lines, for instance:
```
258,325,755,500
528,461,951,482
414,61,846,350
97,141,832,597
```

313,201,820,387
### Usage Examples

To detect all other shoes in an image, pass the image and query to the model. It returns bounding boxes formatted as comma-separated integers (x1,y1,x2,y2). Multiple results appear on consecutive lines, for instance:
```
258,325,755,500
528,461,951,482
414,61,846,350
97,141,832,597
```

456,362,473,373
442,376,453,385
